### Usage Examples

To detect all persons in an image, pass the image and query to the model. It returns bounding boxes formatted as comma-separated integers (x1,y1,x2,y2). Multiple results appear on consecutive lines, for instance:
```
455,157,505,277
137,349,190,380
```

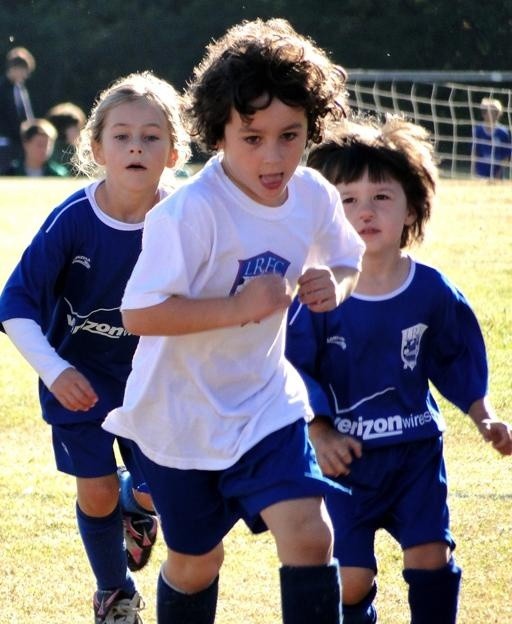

94,14,373,624
1,44,37,148
1,116,71,176
286,106,512,623
44,96,88,177
1,67,194,623
467,95,512,179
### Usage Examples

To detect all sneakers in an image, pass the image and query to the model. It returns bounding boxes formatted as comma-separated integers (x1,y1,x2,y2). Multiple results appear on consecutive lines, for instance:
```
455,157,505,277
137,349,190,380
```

115,464,159,572
92,577,143,624
